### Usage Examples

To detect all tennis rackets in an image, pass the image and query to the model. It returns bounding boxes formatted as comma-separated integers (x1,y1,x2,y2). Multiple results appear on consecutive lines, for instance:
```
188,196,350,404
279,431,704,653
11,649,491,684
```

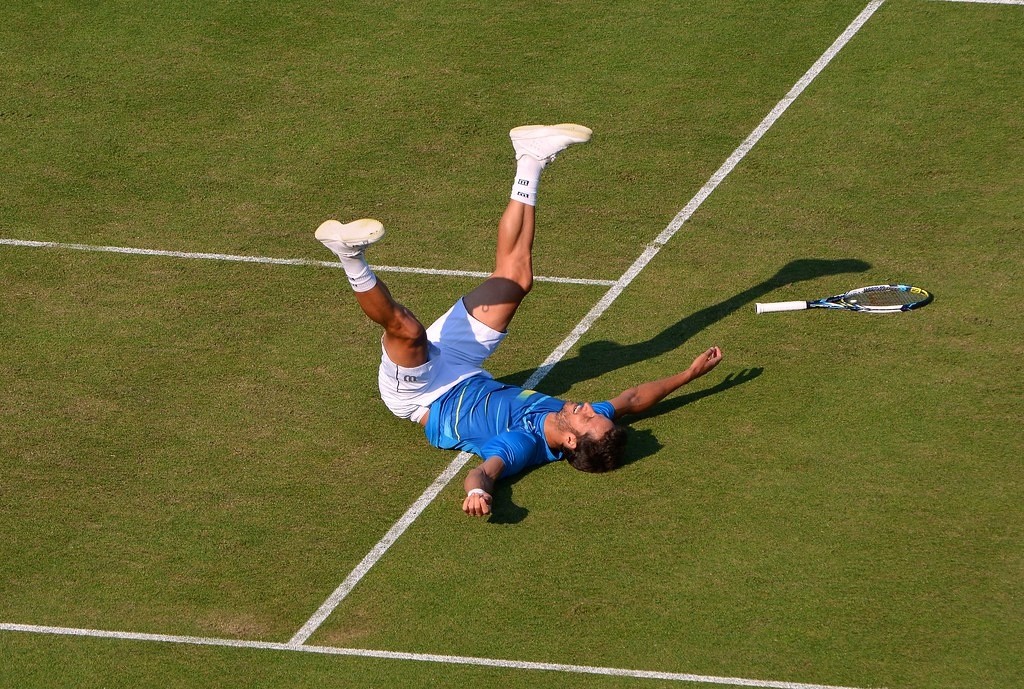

755,284,933,314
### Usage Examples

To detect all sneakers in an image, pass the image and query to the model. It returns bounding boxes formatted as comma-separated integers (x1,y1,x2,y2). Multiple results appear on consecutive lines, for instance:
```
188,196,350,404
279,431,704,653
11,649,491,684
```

509,122,593,161
314,218,386,256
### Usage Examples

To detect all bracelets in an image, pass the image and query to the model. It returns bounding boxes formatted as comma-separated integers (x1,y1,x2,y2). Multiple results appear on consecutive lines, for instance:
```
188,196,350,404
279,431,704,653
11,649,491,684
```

468,488,486,496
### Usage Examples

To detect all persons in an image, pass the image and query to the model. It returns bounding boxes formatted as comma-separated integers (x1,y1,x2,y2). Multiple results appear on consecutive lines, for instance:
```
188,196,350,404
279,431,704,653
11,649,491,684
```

315,124,723,518
